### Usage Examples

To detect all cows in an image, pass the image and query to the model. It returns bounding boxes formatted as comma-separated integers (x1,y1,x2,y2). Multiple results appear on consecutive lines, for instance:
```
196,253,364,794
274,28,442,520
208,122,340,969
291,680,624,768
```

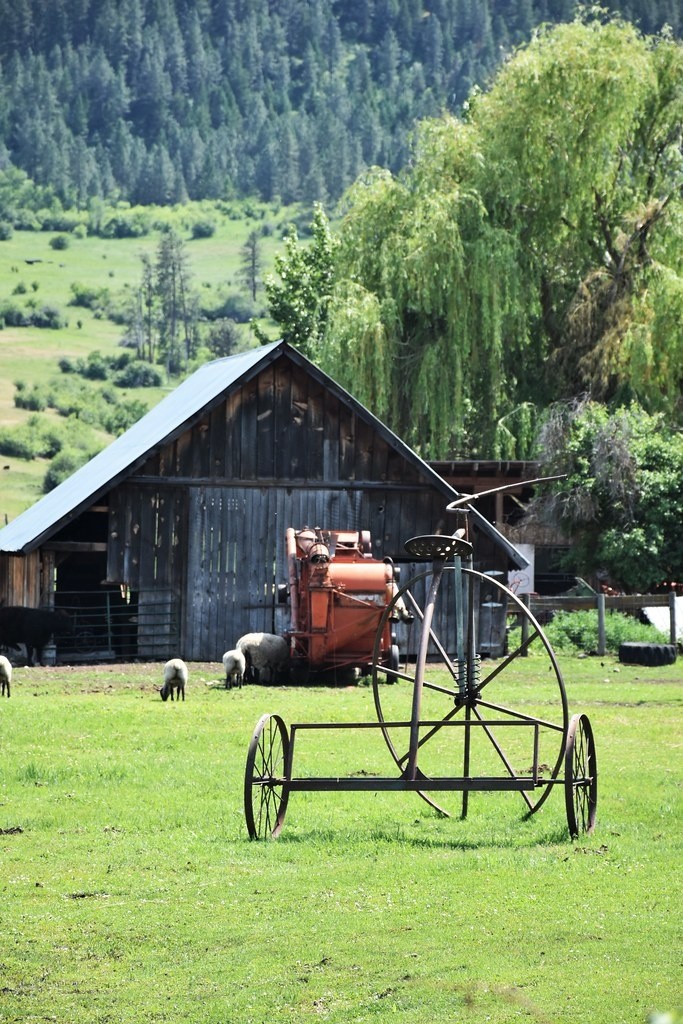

0,606,75,667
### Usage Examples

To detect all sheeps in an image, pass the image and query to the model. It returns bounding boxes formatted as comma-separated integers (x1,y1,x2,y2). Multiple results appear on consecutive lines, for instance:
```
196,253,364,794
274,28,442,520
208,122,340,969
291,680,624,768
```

222,632,289,690
157,659,188,702
0,656,13,697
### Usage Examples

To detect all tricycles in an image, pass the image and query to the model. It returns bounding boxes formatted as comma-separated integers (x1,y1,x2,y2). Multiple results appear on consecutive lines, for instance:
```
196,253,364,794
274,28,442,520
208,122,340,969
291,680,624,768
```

243,473,598,841
